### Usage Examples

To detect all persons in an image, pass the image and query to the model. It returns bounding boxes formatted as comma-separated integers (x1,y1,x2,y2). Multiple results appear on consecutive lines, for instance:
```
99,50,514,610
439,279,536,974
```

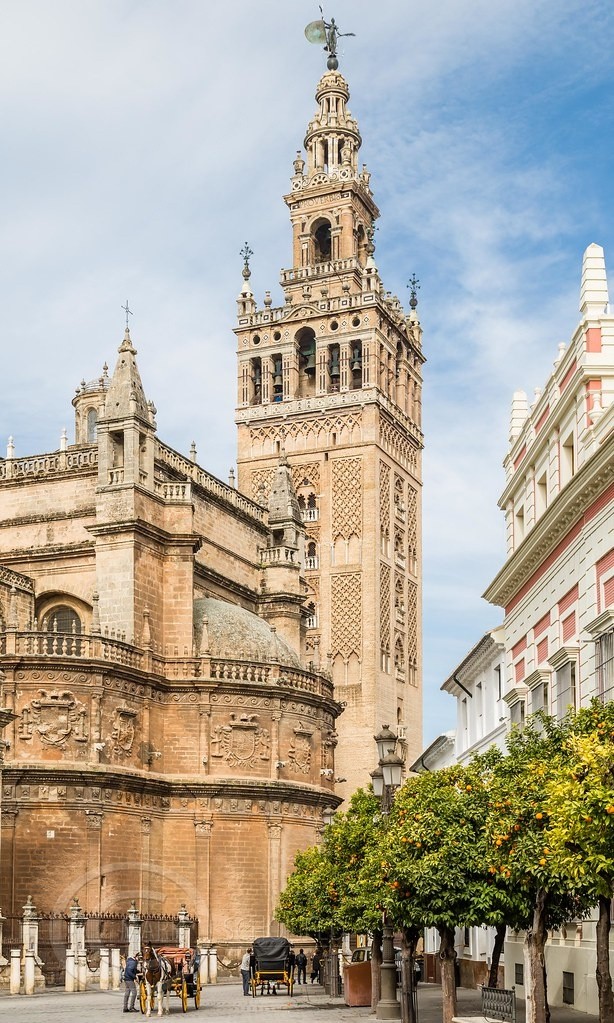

123,952,140,1012
311,950,324,984
288,950,295,984
296,949,307,984
241,948,252,996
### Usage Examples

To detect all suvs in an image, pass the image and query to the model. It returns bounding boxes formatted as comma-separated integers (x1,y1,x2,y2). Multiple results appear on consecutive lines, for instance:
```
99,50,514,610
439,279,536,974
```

350,945,422,987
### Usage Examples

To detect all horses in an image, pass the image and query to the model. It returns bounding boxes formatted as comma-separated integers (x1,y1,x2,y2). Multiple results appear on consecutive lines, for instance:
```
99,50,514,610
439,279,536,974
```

141,941,175,1017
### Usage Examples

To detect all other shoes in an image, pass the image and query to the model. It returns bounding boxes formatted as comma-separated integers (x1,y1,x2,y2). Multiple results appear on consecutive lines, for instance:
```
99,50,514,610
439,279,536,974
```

303,982,307,984
123,1009,130,1012
130,1008,140,1012
244,993,252,996
298,983,301,984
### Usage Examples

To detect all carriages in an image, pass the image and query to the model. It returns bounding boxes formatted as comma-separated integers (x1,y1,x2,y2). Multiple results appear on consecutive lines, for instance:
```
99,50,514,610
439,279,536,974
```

135,940,202,1017
247,936,296,999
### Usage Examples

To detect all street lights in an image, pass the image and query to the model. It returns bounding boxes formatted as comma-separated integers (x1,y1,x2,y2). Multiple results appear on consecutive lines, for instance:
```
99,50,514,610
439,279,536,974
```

367,724,407,1020
316,804,342,997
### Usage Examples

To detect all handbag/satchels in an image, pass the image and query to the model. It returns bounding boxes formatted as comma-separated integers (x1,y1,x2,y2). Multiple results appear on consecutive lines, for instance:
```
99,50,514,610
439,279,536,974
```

310,973,315,978
